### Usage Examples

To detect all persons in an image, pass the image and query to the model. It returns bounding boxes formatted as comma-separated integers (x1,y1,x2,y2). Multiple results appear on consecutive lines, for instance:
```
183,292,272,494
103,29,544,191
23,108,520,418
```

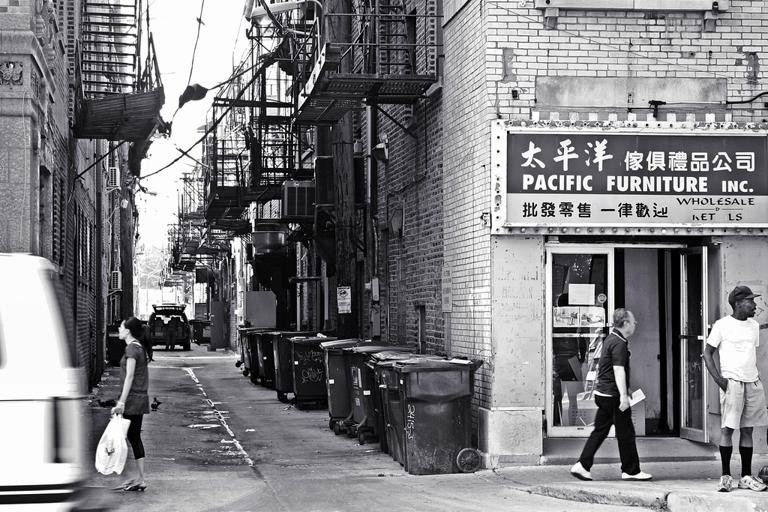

703,286,767,492
114,317,150,492
142,325,154,361
571,309,652,481
166,317,178,350
553,293,587,426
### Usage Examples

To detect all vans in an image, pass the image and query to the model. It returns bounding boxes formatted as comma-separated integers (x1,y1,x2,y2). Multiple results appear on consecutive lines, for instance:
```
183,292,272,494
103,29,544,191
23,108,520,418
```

0,252,88,510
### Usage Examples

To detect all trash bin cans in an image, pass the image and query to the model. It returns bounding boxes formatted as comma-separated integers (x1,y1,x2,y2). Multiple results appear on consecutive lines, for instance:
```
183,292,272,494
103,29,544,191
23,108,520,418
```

235,325,485,475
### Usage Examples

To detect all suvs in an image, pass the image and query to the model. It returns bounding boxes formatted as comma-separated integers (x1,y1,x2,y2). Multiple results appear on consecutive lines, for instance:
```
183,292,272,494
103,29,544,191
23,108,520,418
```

148,303,192,351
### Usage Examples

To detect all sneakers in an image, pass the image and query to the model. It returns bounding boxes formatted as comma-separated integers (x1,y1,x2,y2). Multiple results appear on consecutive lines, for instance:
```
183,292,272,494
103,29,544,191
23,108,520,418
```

570,461,592,480
718,475,733,491
622,471,652,480
738,475,766,491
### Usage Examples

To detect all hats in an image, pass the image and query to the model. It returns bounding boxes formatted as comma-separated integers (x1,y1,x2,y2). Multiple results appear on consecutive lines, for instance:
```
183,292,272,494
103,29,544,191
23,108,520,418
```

729,287,760,303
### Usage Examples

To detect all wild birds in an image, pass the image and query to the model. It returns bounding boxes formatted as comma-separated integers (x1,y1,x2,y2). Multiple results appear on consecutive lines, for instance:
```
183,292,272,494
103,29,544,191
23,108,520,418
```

196,17,205,27
151,396,163,411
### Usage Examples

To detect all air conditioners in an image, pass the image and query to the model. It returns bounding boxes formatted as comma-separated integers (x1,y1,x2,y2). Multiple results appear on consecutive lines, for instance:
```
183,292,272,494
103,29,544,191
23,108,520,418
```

279,180,316,219
110,269,123,290
107,163,122,189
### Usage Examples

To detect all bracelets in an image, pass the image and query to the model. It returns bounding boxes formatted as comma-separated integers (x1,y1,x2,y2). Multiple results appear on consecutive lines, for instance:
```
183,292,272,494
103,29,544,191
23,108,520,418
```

117,401,125,405
